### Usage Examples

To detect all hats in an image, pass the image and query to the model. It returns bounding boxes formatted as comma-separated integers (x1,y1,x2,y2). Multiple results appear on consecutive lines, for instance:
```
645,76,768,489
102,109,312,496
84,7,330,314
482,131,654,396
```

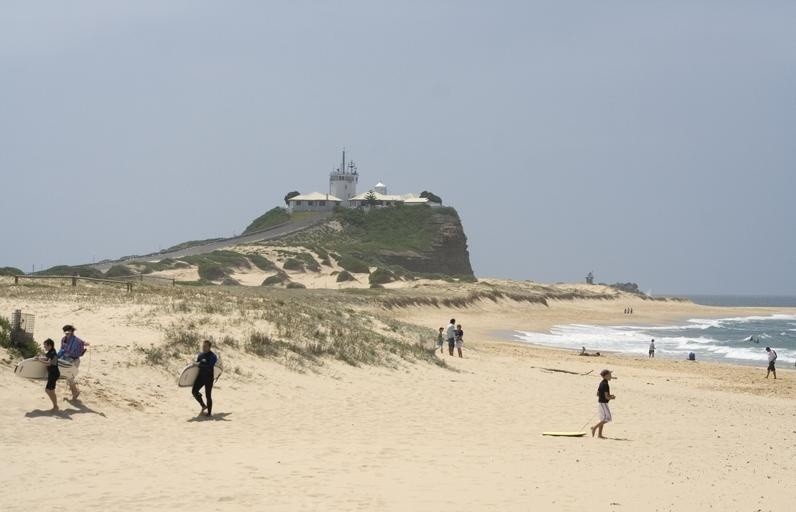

600,368,614,377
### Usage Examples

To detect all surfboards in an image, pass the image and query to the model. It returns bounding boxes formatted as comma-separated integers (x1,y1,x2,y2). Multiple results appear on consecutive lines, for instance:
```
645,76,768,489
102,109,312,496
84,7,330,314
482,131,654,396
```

14,357,78,379
178,364,223,386
542,432,586,436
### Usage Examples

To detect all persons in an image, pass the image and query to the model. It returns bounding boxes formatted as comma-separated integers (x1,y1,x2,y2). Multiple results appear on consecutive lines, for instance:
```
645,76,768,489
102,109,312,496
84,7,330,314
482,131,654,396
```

688,351,696,360
624,308,626,314
455,324,464,358
33,337,60,411
446,318,456,357
765,346,777,379
627,307,630,315
589,368,616,438
56,325,91,398
579,346,600,357
191,340,218,418
630,308,633,314
434,327,444,353
648,339,656,358
